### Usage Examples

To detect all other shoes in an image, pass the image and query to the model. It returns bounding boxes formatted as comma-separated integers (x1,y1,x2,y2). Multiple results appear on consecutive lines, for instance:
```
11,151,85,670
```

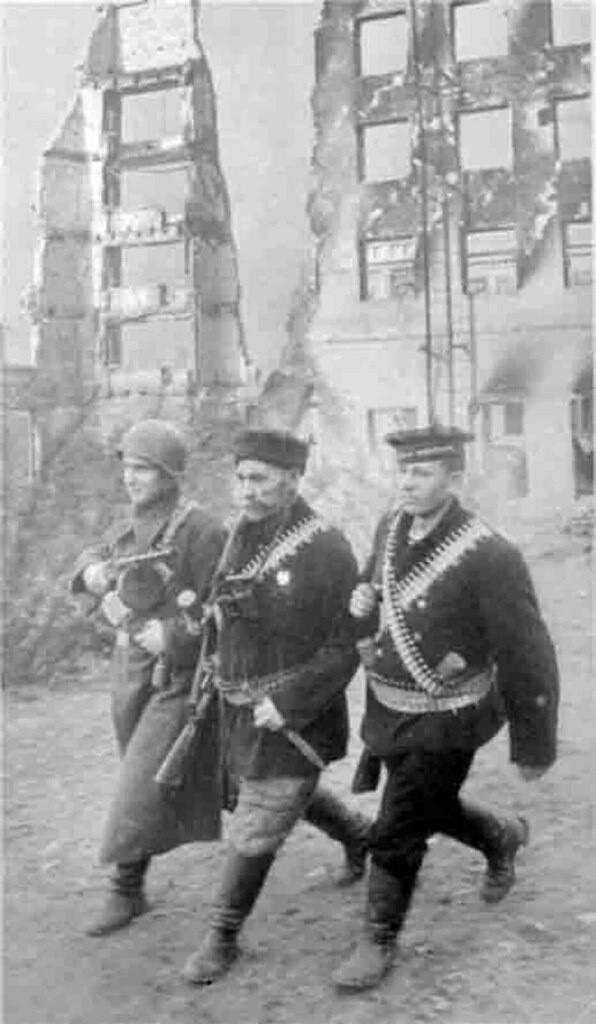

479,818,527,901
330,926,395,988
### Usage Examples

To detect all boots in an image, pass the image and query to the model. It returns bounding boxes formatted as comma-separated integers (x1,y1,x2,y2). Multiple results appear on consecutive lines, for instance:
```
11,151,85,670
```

183,848,276,983
299,791,374,876
88,855,150,936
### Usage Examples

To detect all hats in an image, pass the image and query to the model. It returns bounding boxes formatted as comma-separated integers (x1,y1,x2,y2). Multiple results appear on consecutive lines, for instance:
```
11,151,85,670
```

384,426,476,463
232,430,308,475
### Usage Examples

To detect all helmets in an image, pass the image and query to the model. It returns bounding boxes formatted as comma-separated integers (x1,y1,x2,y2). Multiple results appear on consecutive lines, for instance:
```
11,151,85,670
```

117,420,185,478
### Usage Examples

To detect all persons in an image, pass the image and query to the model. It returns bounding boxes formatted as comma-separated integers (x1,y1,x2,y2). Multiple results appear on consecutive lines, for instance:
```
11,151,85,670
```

67,418,240,939
182,425,376,984
332,420,560,989
